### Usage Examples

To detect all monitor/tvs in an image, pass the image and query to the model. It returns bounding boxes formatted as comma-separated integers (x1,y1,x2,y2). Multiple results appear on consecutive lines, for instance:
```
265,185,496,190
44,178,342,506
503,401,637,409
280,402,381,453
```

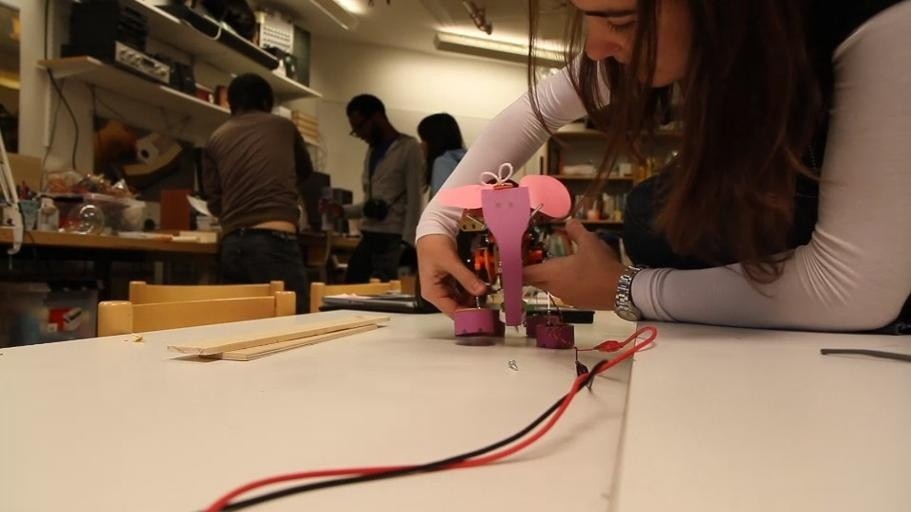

313,171,329,212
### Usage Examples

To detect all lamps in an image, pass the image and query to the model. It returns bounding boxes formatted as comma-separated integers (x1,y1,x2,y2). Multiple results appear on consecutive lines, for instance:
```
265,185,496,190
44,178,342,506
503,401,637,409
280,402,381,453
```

433,30,571,68
312,0,354,30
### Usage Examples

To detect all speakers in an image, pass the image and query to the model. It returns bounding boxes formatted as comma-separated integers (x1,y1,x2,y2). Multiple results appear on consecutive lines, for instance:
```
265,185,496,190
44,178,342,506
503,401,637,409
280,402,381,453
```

174,62,196,95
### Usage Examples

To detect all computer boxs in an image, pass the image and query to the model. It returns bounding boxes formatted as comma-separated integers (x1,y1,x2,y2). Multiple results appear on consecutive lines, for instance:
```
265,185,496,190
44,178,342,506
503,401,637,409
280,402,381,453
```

333,189,352,232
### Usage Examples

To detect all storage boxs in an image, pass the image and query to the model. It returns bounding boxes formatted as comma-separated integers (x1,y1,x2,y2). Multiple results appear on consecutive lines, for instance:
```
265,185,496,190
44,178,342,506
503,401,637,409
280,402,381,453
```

0,288,99,348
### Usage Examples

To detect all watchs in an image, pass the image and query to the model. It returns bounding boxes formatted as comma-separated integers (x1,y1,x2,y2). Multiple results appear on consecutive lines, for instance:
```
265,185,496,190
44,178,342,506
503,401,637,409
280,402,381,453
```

613,263,654,322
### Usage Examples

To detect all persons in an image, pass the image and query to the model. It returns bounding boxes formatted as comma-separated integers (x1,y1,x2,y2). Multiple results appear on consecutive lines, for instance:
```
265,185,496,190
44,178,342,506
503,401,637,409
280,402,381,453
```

317,93,419,288
413,0,911,334
416,112,470,202
202,70,323,315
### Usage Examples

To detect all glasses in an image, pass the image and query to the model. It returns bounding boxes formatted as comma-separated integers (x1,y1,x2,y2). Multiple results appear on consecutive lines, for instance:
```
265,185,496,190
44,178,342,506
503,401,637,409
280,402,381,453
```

350,118,366,137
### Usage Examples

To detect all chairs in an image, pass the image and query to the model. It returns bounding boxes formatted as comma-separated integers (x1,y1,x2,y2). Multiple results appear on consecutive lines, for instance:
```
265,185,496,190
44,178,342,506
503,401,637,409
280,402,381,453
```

308,279,402,314
128,282,285,296
98,290,296,337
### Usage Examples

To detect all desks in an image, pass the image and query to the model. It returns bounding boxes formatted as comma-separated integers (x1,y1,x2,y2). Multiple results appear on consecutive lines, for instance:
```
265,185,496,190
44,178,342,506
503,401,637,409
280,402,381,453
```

1,225,363,285
0,303,911,512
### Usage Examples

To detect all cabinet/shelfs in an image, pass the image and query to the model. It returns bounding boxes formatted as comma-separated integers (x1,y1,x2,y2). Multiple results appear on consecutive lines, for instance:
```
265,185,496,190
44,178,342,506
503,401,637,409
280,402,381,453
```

543,131,680,225
38,0,323,147
